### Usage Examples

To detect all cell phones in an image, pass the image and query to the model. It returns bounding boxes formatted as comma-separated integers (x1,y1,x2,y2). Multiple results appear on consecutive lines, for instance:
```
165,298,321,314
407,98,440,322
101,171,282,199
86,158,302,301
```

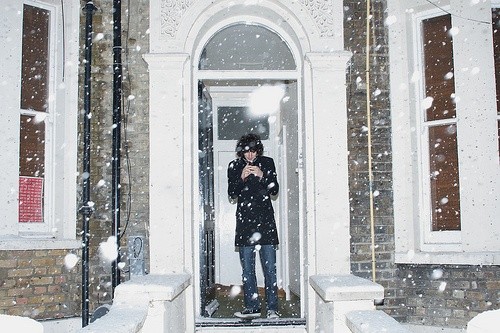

248,161,255,175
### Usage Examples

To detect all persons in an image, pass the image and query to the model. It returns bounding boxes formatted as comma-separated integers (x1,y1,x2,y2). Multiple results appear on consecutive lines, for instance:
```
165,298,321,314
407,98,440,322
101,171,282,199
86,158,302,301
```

227,132,281,318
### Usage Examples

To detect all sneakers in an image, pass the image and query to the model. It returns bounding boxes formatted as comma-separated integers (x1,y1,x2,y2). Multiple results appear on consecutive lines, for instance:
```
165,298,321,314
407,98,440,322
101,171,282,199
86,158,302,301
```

234,308,261,318
267,310,280,319
